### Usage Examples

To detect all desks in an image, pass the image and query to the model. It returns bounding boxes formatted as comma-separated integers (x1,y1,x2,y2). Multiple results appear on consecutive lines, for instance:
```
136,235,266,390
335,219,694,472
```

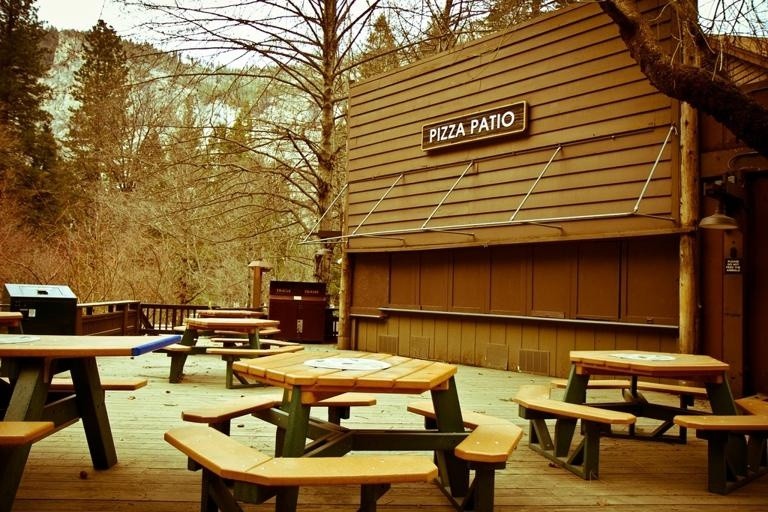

169,310,265,384
0,312,25,334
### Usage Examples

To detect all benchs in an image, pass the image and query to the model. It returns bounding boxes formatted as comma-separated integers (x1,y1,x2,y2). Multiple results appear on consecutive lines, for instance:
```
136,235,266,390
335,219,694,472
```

166,390,282,512
281,449,437,512
312,395,375,427
554,378,712,444
454,408,522,512
407,402,438,431
675,397,768,495
514,386,636,481
0,423,53,488
46,379,147,399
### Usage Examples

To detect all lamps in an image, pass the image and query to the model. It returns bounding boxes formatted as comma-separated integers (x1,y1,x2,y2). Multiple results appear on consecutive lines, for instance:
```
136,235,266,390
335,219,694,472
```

699,182,739,229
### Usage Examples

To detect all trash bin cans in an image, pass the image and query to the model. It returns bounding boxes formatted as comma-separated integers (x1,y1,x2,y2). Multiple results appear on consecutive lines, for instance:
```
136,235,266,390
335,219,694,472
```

4,284,78,335
